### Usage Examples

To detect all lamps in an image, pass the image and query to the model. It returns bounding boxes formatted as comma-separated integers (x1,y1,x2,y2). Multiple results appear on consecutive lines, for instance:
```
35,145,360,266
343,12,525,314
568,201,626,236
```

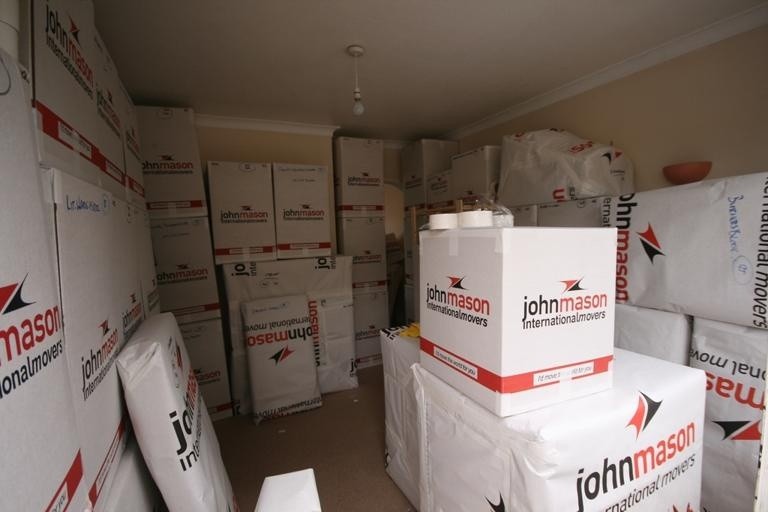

345,44,368,118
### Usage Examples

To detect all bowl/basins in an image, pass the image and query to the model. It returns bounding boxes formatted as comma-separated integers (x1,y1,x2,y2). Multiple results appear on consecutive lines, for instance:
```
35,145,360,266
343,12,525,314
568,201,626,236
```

660,163,713,186
